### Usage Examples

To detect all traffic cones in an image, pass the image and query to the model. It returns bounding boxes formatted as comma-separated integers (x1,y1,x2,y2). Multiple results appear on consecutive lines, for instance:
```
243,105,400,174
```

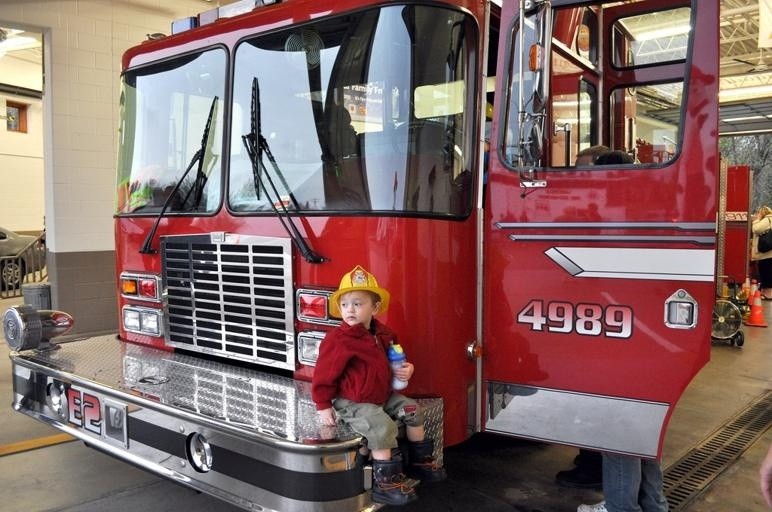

743,289,768,328
739,275,751,301
747,279,759,306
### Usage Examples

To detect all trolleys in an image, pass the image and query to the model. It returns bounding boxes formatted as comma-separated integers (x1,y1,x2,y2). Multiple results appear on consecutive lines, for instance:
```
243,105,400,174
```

710,275,745,347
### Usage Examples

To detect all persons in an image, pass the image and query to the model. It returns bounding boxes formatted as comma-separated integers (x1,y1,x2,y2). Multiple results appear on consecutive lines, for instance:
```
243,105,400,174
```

310,266,449,506
551,143,623,491
572,149,674,511
750,204,772,301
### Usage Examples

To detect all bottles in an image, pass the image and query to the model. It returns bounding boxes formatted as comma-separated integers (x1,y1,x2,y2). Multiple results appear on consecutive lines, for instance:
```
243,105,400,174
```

389,343,408,390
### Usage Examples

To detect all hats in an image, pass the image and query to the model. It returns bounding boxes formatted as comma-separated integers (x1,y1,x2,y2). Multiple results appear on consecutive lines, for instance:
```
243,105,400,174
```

329,265,390,319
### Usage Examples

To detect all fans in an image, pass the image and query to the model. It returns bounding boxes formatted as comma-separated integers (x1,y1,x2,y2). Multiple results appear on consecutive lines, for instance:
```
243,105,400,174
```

711,300,743,340
284,28,326,71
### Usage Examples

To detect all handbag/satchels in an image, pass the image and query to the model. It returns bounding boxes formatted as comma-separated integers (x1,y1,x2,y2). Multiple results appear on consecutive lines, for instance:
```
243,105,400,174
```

758,216,772,253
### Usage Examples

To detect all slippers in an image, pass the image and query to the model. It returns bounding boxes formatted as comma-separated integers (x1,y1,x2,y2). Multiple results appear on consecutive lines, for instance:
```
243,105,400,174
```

761,295,772,300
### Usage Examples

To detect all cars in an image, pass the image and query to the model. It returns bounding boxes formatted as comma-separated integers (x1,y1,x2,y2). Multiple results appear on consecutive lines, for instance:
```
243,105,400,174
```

0,228,45,291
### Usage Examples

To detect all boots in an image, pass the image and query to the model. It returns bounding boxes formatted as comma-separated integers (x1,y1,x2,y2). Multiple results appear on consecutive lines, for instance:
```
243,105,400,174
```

408,439,447,482
372,457,417,505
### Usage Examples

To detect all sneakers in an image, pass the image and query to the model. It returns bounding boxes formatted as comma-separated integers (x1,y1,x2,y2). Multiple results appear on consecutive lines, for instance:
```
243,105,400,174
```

577,501,608,512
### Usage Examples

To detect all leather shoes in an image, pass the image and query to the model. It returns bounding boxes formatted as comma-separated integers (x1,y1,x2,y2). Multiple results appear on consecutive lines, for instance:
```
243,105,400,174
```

556,450,602,489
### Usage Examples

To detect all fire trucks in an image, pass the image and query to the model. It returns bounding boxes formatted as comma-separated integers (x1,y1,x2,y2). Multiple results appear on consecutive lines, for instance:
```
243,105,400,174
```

2,0,750,512
